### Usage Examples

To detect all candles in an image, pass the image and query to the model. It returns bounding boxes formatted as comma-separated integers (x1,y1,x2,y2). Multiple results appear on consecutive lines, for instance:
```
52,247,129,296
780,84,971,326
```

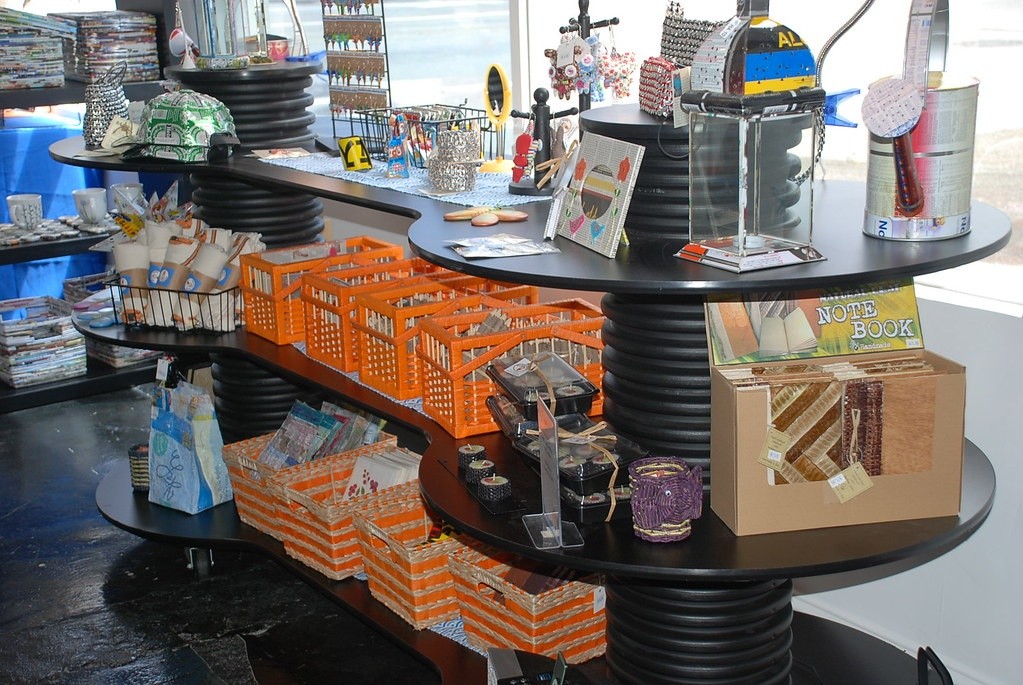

458,444,486,470
466,460,496,483
478,473,511,502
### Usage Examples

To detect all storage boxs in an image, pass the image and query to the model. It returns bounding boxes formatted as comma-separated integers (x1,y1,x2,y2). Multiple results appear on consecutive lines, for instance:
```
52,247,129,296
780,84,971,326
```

239,235,403,345
103,278,243,335
532,467,635,525
63,272,165,368
353,493,478,629
222,433,397,541
485,351,600,419
266,447,422,580
513,414,650,495
448,545,607,664
356,276,538,400
418,297,606,439
302,256,448,374
486,396,529,442
0,296,87,388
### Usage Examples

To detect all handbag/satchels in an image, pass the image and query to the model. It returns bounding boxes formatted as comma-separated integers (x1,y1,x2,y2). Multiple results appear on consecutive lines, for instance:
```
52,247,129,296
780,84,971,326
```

148,382,235,515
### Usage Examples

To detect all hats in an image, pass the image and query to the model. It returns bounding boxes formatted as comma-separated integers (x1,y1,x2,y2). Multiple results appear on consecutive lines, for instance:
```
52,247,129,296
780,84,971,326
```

111,89,243,163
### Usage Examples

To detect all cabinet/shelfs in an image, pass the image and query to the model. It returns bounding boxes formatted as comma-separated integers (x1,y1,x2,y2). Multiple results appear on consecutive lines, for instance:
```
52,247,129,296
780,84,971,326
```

48,135,1011,685
0,1,157,411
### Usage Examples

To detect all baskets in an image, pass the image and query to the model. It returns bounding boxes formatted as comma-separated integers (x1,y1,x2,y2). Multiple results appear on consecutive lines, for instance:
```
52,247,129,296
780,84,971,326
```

240,235,607,438
221,429,607,667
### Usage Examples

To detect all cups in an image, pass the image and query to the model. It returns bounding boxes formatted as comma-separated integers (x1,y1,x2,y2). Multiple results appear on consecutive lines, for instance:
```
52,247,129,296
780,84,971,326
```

72,188,107,223
110,183,143,213
7,194,43,230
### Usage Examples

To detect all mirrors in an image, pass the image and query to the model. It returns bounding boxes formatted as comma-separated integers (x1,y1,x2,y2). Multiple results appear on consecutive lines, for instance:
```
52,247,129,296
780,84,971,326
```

488,67,504,118
